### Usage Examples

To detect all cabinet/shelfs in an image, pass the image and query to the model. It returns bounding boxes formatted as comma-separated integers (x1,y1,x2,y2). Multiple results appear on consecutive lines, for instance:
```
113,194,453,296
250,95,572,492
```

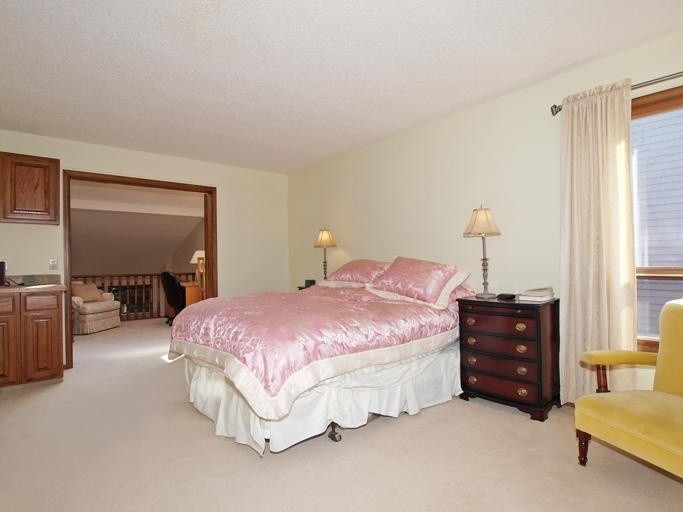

0,151,60,226
0,284,67,387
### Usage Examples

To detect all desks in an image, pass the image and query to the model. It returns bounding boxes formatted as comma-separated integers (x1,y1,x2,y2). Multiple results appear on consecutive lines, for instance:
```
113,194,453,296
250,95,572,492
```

164,282,200,317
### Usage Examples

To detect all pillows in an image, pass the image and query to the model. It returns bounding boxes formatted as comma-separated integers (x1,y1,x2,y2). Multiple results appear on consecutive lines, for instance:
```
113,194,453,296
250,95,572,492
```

365,256,471,310
71,282,98,302
315,259,393,288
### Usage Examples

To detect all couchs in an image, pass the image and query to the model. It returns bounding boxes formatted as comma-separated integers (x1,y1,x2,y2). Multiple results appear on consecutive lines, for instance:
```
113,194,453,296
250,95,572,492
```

71,280,121,335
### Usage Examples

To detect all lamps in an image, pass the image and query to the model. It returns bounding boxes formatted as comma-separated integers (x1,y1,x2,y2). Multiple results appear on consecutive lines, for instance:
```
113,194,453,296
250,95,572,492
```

188,250,205,300
313,224,336,280
462,203,501,298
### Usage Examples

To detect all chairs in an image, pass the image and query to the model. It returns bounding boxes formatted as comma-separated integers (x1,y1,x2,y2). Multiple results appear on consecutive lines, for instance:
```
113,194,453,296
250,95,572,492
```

572,299,682,480
159,271,185,327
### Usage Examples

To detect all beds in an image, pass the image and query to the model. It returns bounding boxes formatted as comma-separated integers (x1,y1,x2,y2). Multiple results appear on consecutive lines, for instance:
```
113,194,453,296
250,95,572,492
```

167,283,477,457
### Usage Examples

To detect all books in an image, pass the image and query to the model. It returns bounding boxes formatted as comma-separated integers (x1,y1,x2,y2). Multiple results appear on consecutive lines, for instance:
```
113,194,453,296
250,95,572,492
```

522,287,553,296
519,295,553,302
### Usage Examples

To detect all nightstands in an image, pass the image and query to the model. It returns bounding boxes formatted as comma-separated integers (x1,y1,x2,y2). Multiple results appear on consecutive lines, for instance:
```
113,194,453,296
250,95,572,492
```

456,295,562,422
297,285,305,290
